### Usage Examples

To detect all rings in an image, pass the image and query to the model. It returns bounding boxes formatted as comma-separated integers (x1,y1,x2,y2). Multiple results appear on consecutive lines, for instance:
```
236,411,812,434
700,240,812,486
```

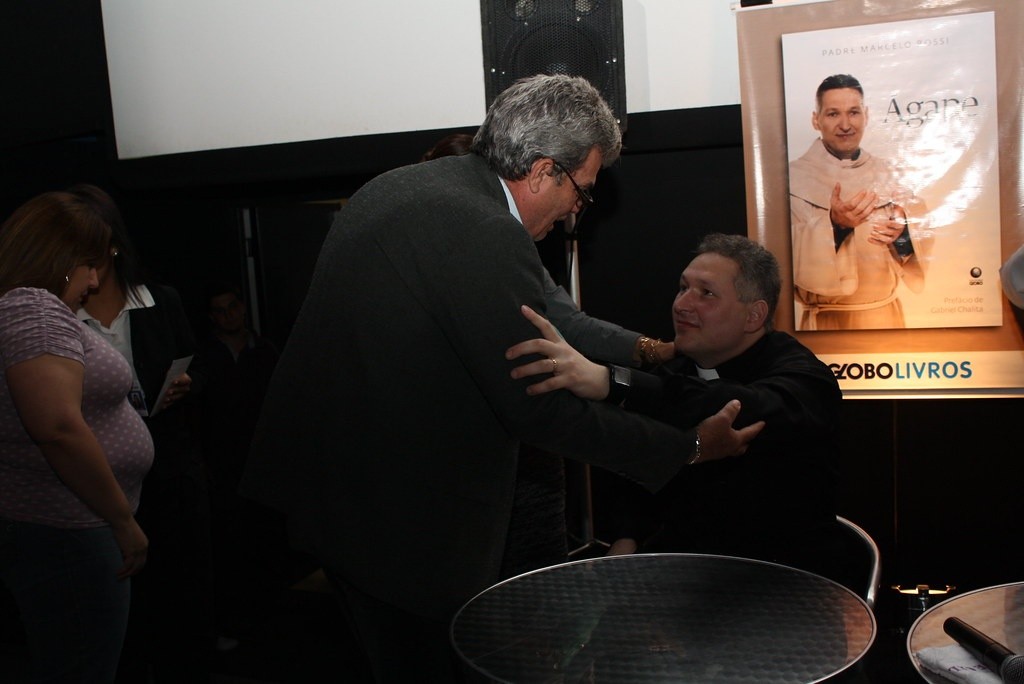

551,358,557,373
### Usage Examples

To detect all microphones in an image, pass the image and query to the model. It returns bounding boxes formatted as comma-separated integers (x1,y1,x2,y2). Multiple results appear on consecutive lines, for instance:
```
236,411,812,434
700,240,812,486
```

943,617,1024,684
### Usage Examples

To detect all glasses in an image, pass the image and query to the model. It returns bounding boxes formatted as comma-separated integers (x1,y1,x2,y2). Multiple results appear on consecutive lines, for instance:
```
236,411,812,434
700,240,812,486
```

536,155,593,209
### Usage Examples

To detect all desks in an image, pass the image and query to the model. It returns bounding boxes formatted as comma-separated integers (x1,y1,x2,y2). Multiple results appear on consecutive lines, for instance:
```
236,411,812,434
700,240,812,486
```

907,582,1024,684
449,553,877,684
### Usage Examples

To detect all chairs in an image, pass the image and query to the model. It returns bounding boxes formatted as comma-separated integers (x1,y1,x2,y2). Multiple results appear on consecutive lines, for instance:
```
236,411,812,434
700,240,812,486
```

837,515,880,613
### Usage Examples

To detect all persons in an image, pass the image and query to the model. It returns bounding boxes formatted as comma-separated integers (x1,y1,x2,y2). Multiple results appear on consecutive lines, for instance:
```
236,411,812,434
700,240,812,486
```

193,283,282,651
133,394,144,411
64,184,211,684
506,232,847,574
788,74,934,330
236,75,766,684
419,134,554,243
0,191,155,684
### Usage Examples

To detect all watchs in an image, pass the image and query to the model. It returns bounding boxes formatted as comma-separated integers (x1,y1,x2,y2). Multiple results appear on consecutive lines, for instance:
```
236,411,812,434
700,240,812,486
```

600,362,634,403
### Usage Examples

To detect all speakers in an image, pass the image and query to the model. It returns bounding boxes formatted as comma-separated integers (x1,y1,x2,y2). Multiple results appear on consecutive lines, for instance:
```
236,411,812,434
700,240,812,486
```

480,0,627,133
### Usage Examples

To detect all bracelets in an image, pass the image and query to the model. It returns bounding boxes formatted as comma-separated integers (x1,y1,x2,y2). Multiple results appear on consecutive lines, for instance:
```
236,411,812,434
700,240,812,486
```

639,337,663,366
687,434,704,465
